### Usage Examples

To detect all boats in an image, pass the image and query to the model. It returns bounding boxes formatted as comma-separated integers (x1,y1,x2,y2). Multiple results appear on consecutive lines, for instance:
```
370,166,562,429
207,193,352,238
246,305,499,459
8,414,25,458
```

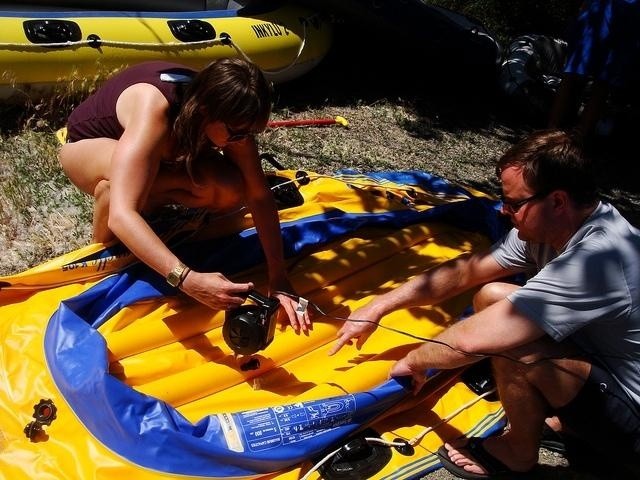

0,0,333,108
2,171,508,479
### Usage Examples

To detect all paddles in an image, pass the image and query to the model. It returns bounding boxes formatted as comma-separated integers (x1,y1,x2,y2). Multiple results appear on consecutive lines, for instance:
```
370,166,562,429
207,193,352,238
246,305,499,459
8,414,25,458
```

56,115,350,147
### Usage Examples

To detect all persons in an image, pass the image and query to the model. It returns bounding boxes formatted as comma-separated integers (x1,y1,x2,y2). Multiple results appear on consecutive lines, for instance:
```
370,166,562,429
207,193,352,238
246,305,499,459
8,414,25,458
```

326,129,640,480
548,0,639,149
60,56,314,331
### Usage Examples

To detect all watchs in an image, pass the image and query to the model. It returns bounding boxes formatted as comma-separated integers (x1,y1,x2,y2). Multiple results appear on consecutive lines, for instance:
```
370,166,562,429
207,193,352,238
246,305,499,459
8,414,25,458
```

165,260,188,288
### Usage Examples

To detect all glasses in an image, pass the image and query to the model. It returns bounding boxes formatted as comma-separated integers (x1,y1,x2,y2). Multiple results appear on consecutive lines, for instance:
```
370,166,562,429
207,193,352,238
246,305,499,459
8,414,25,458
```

223,119,257,144
500,193,544,215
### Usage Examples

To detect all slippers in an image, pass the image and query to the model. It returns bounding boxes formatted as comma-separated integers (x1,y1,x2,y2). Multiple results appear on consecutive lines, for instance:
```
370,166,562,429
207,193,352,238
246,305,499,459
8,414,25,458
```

539,419,574,456
437,436,541,480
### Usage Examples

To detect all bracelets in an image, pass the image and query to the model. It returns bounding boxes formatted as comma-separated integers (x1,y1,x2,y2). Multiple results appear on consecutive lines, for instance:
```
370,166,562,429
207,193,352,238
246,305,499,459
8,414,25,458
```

577,6,584,14
